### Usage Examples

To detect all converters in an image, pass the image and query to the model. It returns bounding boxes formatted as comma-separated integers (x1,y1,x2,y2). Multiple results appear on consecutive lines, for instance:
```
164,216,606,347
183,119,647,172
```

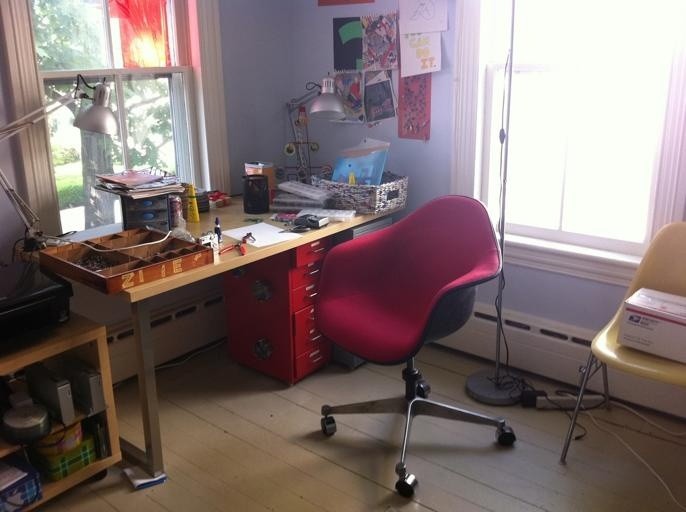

521,390,547,409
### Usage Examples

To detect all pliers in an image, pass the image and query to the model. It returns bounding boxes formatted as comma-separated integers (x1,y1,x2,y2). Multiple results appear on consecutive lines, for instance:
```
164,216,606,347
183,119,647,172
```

219,241,247,255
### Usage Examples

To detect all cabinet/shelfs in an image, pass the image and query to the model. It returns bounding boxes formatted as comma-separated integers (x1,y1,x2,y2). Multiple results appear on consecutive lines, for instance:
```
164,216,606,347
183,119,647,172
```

0,313,121,512
331,216,393,371
225,235,334,386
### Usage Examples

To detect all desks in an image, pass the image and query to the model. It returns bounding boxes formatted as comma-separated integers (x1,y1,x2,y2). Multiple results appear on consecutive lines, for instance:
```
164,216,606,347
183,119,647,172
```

14,195,405,476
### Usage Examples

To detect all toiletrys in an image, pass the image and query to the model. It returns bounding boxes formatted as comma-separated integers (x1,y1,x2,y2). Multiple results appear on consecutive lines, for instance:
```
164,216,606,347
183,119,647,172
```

186,184,200,224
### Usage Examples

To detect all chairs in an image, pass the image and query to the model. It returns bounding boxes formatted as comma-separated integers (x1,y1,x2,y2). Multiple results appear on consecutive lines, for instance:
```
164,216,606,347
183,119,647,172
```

313,194,516,497
560,222,686,463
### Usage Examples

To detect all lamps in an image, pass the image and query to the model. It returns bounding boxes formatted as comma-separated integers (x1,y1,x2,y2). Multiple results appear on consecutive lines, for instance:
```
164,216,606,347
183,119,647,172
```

286,77,344,184
0,74,119,251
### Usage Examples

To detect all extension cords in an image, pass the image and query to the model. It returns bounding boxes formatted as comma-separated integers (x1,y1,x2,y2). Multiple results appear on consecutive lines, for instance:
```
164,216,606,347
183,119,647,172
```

536,395,605,409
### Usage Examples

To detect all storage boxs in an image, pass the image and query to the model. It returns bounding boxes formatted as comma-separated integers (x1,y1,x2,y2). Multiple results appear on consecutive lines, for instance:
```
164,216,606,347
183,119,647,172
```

38,228,213,294
616,287,686,363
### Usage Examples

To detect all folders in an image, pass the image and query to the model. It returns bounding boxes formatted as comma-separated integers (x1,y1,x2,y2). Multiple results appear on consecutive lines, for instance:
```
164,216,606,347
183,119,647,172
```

330,136,391,186
